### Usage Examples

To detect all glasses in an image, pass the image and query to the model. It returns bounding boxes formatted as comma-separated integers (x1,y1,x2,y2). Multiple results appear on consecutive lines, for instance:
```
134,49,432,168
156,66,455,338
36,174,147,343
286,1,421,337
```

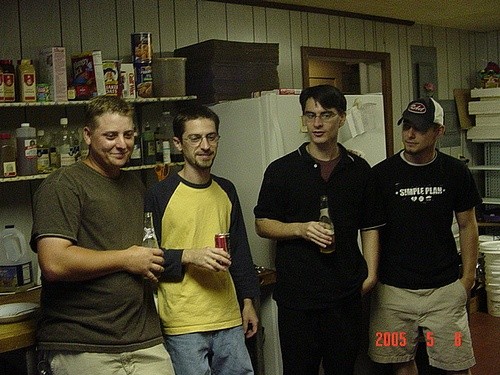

182,133,222,147
301,109,340,120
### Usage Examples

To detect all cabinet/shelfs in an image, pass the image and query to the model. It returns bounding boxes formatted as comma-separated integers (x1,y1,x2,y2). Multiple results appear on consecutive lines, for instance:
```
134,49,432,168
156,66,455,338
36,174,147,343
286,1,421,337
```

467,140,500,205
0,92,205,182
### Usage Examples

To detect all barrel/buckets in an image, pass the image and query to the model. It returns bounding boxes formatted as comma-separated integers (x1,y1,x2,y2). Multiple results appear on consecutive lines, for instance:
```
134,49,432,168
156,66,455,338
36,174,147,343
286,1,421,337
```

480,240,500,318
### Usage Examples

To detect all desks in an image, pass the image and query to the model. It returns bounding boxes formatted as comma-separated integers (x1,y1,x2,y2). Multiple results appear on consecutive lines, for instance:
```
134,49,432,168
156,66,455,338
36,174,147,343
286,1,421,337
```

0,264,276,375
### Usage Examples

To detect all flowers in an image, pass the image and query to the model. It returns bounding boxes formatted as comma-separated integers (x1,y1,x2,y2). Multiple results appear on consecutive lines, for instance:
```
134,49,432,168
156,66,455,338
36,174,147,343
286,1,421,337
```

426,83,436,98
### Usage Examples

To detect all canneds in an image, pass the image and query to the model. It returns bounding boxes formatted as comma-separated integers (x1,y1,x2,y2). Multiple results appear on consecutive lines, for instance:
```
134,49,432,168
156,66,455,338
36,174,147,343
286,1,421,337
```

214,233,232,267
131,31,153,98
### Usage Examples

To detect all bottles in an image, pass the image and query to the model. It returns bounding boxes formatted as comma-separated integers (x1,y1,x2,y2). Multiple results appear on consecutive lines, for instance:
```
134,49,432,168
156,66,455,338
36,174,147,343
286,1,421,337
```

318,193,335,254
141,212,162,279
0,225,39,293
0,111,185,178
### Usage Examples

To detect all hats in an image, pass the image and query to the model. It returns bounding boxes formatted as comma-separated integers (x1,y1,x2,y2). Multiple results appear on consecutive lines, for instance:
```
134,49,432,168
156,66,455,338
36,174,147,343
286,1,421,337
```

396,95,444,131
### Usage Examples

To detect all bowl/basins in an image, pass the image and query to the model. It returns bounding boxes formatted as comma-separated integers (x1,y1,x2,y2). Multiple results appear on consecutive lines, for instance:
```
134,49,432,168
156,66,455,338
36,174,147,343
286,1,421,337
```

0,303,38,323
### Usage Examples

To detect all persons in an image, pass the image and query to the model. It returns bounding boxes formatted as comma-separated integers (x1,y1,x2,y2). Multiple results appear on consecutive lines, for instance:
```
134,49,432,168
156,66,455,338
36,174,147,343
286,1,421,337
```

367,97,483,375
253,84,387,375
28,96,175,375
145,106,259,375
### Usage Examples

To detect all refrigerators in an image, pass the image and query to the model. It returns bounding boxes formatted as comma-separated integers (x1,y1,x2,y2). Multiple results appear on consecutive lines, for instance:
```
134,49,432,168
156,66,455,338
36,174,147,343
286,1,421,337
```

208,92,387,375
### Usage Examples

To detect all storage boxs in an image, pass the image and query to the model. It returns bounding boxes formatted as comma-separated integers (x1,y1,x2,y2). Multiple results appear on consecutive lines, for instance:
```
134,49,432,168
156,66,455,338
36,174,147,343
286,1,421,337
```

467,89,500,139
0,45,136,101
